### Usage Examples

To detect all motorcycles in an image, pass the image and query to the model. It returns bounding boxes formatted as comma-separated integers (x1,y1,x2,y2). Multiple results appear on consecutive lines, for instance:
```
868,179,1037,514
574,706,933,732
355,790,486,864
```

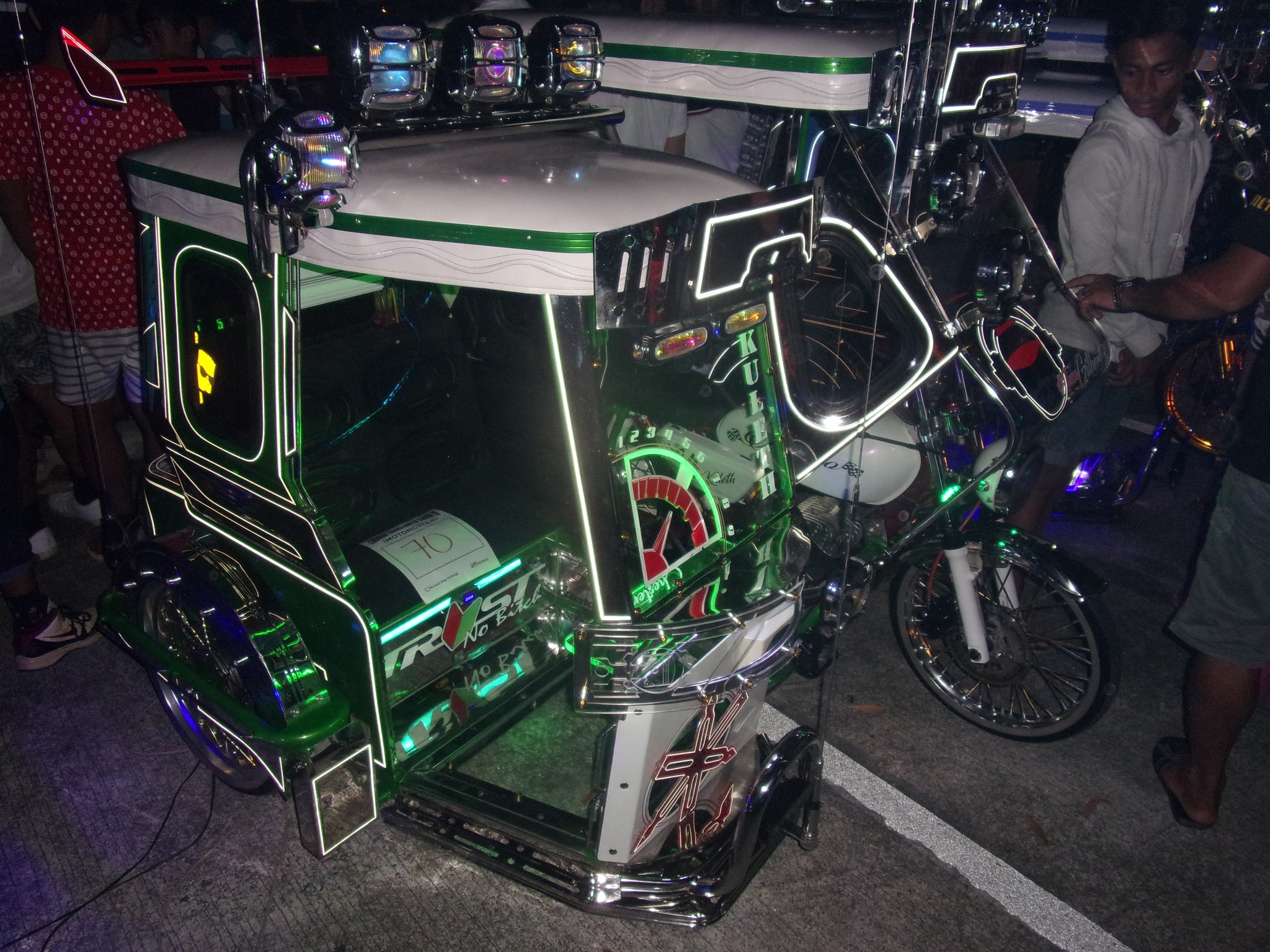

425,0,1270,746
94,0,827,931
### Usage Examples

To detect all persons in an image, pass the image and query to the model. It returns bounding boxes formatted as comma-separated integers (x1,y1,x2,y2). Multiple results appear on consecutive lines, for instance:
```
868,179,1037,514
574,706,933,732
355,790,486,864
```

1009,1,1212,596
1064,163,1270,831
589,86,749,174
0,0,188,670
130,0,275,136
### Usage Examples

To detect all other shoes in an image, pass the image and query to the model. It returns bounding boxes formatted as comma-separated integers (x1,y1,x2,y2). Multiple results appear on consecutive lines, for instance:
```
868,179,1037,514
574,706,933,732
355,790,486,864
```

1031,545,1103,595
50,492,103,529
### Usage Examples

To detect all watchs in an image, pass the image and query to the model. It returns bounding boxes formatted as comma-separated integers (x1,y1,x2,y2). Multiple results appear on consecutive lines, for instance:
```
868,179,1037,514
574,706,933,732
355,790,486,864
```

1112,276,1141,309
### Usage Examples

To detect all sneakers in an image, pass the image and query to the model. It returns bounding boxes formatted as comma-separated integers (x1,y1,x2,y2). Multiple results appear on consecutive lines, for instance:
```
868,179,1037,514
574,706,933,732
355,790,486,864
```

15,602,103,671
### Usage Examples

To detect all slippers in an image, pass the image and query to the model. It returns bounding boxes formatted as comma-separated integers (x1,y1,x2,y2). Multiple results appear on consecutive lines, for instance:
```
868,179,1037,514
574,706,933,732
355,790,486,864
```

1153,738,1212,829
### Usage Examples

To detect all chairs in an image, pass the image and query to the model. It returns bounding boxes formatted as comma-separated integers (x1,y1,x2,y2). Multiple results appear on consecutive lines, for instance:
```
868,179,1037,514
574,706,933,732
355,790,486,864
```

285,308,583,761
